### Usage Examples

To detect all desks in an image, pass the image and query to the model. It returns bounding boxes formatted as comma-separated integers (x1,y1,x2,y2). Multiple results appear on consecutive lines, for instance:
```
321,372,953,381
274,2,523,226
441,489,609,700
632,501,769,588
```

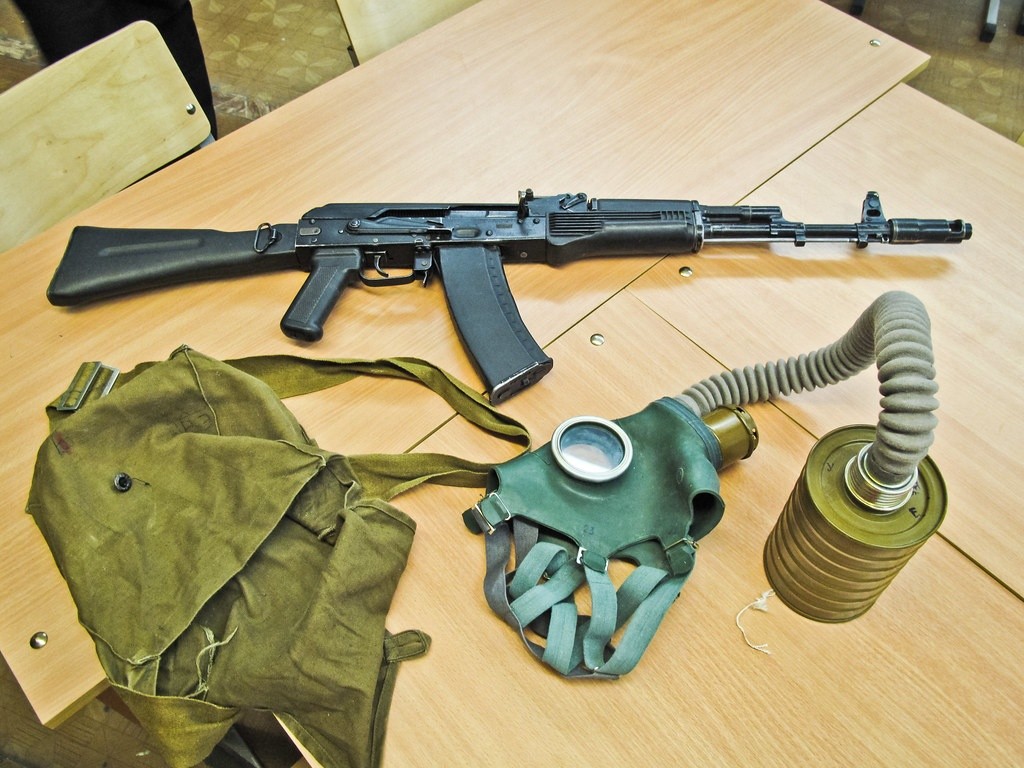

273,289,1024,768
624,82,1024,600
0,0,931,730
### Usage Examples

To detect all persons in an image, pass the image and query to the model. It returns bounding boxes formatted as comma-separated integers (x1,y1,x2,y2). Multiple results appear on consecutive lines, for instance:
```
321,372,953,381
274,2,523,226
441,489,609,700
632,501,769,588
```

8,0,216,186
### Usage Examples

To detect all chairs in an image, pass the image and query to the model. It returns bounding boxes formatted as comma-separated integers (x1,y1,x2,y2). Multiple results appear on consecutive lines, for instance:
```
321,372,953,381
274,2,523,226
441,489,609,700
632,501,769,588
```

335,0,481,67
0,20,216,254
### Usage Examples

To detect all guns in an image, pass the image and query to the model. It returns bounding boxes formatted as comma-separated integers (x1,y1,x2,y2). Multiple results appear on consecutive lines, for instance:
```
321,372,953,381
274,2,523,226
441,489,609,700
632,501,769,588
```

46,187,972,407
